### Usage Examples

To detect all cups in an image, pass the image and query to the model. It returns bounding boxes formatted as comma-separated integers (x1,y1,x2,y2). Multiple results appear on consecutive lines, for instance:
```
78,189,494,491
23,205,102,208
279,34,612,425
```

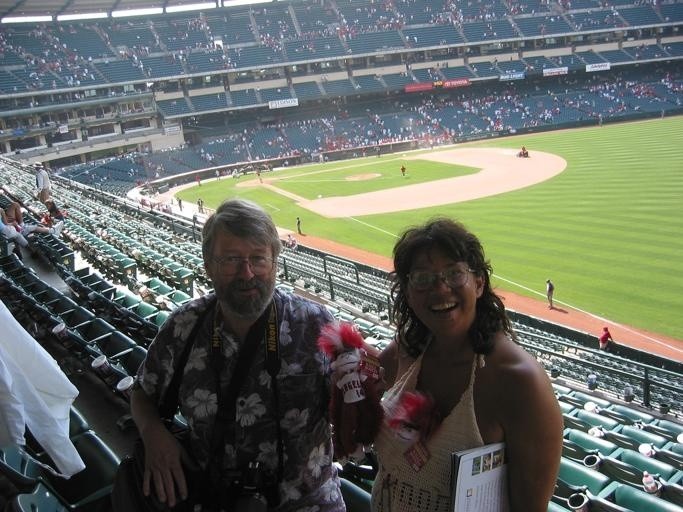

676,432,683,443
137,286,148,298
90,355,115,387
50,323,71,349
584,401,599,415
586,375,595,383
116,374,133,402
638,443,652,457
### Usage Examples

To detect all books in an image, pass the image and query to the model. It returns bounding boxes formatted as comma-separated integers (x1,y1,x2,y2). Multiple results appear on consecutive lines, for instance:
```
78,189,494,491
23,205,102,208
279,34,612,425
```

449,442,507,511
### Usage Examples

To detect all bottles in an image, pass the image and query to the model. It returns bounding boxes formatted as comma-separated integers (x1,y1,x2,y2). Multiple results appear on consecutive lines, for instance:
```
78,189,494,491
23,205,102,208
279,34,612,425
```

641,471,660,497
622,382,632,395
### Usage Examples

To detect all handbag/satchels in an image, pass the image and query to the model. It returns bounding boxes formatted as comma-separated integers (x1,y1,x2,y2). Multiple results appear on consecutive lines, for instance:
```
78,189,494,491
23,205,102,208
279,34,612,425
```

112,417,281,512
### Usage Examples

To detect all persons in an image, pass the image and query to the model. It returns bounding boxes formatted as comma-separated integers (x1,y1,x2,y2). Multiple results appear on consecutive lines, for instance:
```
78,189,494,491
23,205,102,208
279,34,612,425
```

338,215,562,512
599,326,613,351
0,0,683,257
545,279,555,309
563,217,566,218
130,201,348,512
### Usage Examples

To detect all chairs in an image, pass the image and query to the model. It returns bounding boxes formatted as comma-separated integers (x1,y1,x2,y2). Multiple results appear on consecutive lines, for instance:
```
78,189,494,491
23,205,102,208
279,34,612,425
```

284,1,682,160
0,0,284,214
275,241,682,512
0,212,208,512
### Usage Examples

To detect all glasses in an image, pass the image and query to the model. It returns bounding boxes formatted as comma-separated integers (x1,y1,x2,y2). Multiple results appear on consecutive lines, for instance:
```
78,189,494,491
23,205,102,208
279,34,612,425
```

407,265,479,291
210,256,273,276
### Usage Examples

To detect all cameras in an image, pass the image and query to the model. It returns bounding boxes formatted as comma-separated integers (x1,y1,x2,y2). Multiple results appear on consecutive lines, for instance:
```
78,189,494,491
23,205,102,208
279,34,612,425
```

217,462,278,512
